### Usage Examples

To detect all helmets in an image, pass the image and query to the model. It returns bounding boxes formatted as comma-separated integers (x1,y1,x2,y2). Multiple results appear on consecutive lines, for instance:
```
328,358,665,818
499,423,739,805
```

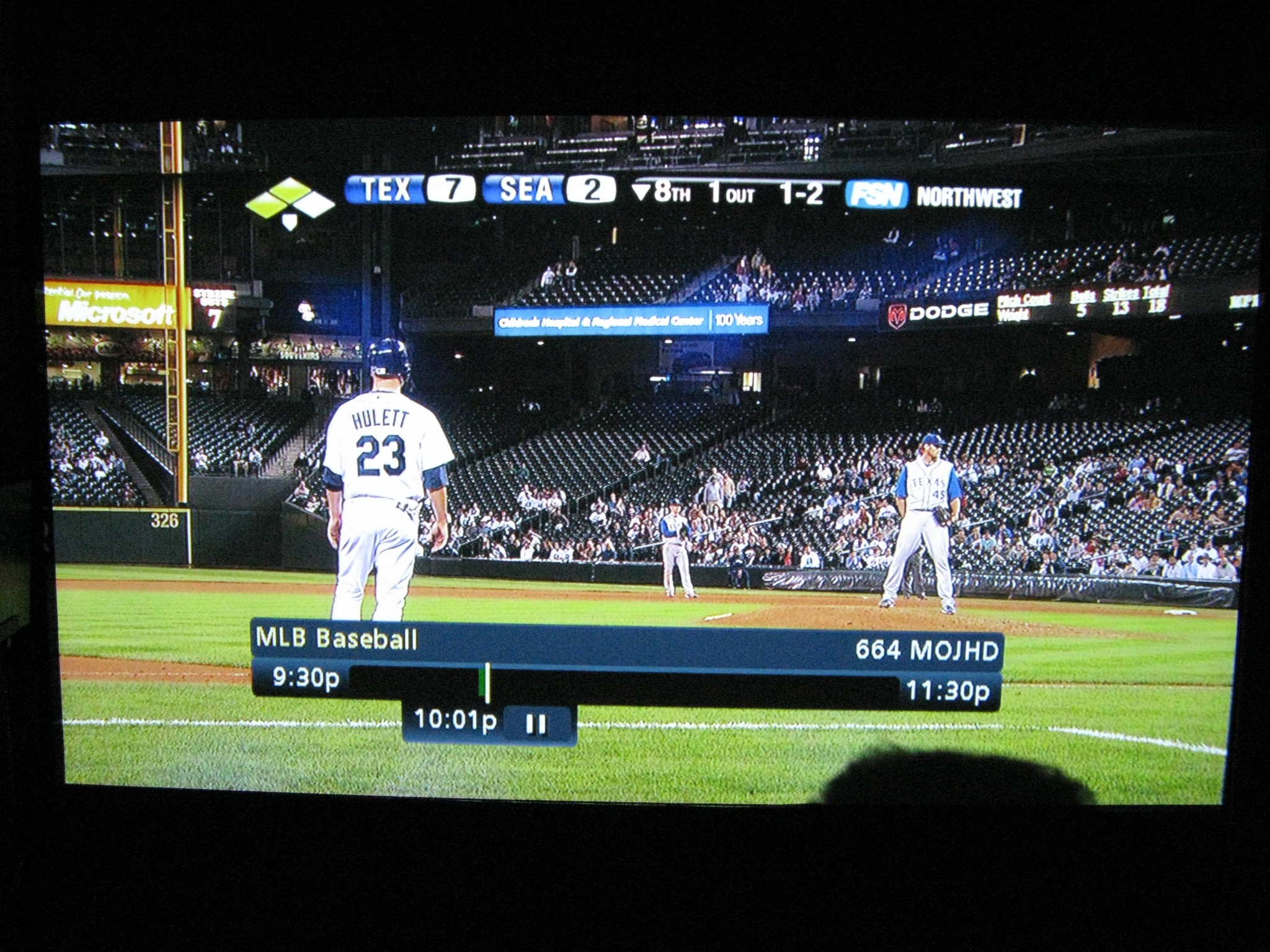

367,338,412,382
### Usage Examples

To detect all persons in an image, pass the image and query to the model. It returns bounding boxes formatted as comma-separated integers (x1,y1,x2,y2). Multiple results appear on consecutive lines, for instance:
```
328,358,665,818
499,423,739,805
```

660,498,699,600
50,113,1260,611
322,339,455,622
879,434,961,616
818,742,1099,952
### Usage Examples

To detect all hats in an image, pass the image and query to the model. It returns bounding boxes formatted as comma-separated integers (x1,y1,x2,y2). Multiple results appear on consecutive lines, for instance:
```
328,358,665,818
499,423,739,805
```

921,434,946,446
670,498,684,506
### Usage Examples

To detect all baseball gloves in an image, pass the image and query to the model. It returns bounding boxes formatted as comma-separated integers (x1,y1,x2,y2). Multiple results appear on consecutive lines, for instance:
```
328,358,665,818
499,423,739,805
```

932,505,952,527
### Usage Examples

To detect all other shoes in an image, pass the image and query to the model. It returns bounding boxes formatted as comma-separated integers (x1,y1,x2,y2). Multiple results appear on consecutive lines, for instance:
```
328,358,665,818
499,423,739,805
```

684,593,698,599
918,592,927,600
904,593,910,599
665,591,674,597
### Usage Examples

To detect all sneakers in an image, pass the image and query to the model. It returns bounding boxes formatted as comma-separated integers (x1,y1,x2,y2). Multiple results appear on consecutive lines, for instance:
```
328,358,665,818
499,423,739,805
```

879,600,893,607
941,605,956,614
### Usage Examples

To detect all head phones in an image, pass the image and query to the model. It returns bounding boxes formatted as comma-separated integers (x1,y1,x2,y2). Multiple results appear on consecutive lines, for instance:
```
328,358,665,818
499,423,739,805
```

390,337,410,376
